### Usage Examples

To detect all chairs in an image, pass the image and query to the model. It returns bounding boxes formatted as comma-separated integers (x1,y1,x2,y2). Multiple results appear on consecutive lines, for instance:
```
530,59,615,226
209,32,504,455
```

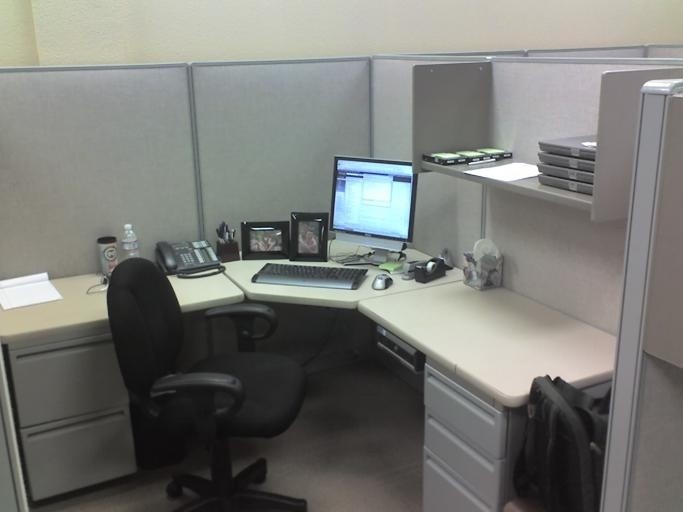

108,258,308,512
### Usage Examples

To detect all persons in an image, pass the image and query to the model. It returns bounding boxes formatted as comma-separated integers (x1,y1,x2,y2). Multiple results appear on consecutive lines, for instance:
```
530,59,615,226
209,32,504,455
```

306,229,314,243
301,221,309,243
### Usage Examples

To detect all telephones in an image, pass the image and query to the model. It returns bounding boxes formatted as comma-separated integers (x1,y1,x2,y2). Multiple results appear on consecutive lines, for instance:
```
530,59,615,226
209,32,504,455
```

155,239,220,275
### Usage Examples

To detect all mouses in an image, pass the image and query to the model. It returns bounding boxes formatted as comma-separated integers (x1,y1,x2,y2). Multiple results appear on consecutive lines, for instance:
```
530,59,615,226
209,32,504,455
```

373,273,392,291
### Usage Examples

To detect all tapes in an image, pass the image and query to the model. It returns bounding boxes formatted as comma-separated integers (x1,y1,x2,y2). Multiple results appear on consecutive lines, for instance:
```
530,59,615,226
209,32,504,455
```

426,261,437,272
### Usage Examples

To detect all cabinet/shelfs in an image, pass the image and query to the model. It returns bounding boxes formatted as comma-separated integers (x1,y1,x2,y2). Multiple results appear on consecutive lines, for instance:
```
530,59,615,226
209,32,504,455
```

420,357,612,511
8,331,141,502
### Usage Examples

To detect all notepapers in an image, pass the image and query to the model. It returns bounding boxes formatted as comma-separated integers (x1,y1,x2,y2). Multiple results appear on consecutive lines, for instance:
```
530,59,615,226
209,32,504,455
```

379,261,403,272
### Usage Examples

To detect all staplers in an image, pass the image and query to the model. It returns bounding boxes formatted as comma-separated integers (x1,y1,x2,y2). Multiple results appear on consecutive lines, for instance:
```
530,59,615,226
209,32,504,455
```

402,261,426,280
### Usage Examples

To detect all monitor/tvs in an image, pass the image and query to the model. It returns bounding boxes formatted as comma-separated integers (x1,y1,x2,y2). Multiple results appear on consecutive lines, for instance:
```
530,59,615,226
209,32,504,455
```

329,153,416,262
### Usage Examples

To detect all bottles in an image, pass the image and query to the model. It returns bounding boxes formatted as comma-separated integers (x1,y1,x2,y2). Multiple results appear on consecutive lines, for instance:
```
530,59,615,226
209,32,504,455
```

122,223,140,262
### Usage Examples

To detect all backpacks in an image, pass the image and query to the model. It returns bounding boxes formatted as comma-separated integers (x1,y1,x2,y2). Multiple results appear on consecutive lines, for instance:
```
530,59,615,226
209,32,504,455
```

514,374,609,509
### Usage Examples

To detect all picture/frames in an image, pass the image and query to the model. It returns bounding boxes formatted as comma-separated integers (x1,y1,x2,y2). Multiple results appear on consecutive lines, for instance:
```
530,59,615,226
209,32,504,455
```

241,212,331,261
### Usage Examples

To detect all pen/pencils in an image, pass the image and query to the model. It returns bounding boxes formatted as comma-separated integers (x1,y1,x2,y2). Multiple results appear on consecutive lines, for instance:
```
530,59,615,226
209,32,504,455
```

462,248,478,281
216,222,236,244
468,158,497,165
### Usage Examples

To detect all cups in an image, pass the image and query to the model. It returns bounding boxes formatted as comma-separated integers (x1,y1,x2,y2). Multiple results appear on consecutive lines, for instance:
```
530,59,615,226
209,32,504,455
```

97,236,118,277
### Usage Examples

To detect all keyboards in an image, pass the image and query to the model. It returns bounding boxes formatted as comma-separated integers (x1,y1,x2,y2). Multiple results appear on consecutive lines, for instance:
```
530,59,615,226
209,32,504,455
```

253,262,368,292
